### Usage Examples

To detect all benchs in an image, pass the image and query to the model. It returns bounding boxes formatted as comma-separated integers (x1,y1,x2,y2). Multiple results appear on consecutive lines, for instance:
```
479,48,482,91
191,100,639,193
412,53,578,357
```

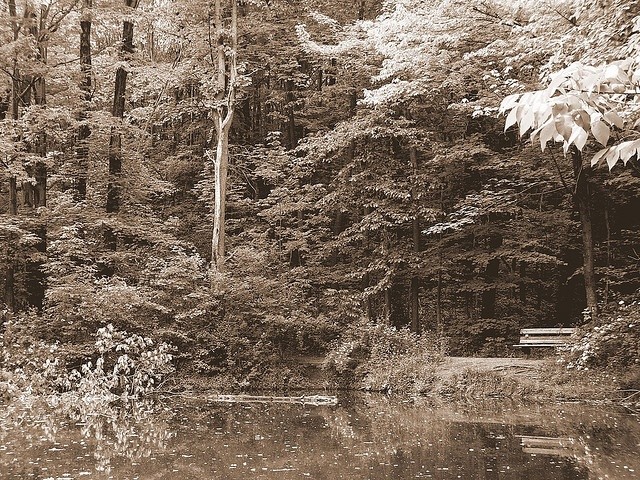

515,435,576,455
512,328,583,360
